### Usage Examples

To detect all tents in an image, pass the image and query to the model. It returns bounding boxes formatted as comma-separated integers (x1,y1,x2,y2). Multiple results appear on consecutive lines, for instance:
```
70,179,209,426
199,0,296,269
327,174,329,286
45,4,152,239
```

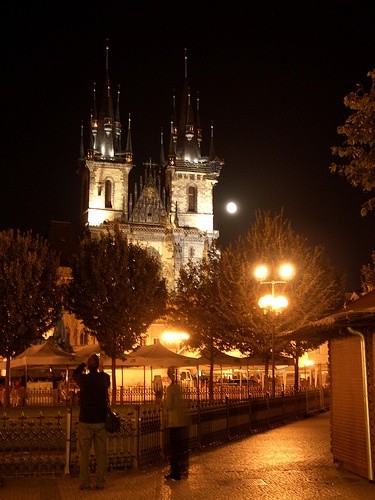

0,340,295,407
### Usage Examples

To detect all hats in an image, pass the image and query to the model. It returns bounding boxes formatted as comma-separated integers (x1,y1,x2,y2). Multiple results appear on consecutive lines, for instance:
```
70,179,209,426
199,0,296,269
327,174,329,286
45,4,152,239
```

87,354,99,368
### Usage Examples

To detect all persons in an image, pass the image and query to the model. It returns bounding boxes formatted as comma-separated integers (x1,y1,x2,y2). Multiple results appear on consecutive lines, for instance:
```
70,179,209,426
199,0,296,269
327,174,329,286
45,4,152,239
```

72,354,112,490
161,363,193,481
152,375,165,399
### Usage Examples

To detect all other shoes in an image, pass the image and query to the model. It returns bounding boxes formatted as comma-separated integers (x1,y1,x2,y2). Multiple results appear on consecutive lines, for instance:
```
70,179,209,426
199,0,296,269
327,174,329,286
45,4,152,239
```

179,471,188,478
96,484,105,490
79,484,91,489
164,473,181,480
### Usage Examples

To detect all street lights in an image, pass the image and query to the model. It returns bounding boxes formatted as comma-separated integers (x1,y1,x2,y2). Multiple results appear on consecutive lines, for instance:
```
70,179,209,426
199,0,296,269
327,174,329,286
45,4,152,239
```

252,262,296,398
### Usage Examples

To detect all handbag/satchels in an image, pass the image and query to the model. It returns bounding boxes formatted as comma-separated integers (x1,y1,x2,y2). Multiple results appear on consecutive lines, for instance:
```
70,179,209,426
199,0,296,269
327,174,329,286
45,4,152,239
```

105,408,121,434
